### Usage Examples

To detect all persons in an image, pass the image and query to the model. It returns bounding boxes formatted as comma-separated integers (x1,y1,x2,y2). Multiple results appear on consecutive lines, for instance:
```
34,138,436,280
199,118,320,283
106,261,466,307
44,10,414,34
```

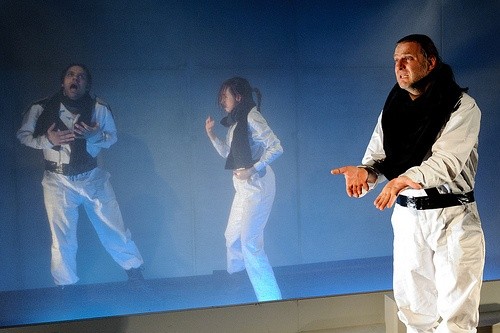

17,63,148,293
331,33,486,333
205,77,288,301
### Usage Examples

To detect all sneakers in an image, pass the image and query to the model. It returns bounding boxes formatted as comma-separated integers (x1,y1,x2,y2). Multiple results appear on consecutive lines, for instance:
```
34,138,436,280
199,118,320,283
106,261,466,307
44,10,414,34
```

57,286,86,300
219,274,249,292
127,270,158,294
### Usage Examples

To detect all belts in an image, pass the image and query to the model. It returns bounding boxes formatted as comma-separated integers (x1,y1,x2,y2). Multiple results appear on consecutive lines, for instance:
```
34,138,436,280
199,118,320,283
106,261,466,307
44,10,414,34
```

394,188,475,211
44,157,98,176
